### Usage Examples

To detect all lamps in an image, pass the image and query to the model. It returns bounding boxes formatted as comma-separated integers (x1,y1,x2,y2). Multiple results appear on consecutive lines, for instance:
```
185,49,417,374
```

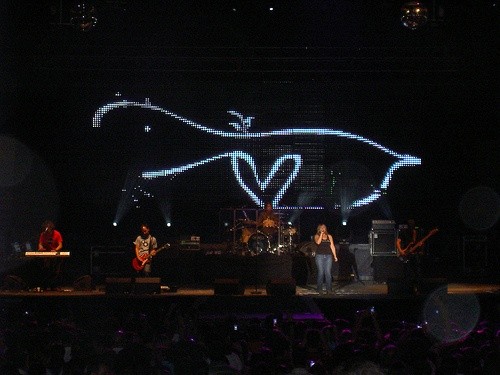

70,0,100,32
399,0,445,32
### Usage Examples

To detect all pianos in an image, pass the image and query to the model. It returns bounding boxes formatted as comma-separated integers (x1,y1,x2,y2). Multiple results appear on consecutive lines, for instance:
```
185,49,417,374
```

24,251,70,293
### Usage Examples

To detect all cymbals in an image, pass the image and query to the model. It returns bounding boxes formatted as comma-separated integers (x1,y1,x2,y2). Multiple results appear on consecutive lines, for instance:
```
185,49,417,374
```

244,220,259,223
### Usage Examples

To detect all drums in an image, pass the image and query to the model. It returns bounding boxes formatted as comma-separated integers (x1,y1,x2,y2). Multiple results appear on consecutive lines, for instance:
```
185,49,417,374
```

282,228,296,235
241,224,257,243
262,218,275,233
246,231,270,255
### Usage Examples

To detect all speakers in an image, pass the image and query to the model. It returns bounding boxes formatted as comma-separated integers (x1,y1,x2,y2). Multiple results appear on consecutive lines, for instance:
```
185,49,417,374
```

135,277,161,295
104,277,133,294
213,279,241,295
419,278,448,296
387,277,417,294
269,278,296,295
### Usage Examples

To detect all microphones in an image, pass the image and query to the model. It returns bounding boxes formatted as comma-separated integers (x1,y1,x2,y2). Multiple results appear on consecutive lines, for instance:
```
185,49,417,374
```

44,226,49,232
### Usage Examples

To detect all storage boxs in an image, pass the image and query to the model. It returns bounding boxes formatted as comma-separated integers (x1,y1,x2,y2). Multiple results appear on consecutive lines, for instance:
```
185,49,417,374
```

369,231,398,256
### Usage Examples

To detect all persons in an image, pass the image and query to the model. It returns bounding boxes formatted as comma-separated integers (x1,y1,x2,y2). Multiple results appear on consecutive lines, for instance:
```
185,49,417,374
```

1,305,499,375
37,218,64,289
315,223,338,293
259,202,281,245
132,222,158,277
396,219,425,254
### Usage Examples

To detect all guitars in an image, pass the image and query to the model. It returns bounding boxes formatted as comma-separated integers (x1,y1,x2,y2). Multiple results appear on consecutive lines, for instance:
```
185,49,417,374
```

131,242,172,272
397,228,438,264
